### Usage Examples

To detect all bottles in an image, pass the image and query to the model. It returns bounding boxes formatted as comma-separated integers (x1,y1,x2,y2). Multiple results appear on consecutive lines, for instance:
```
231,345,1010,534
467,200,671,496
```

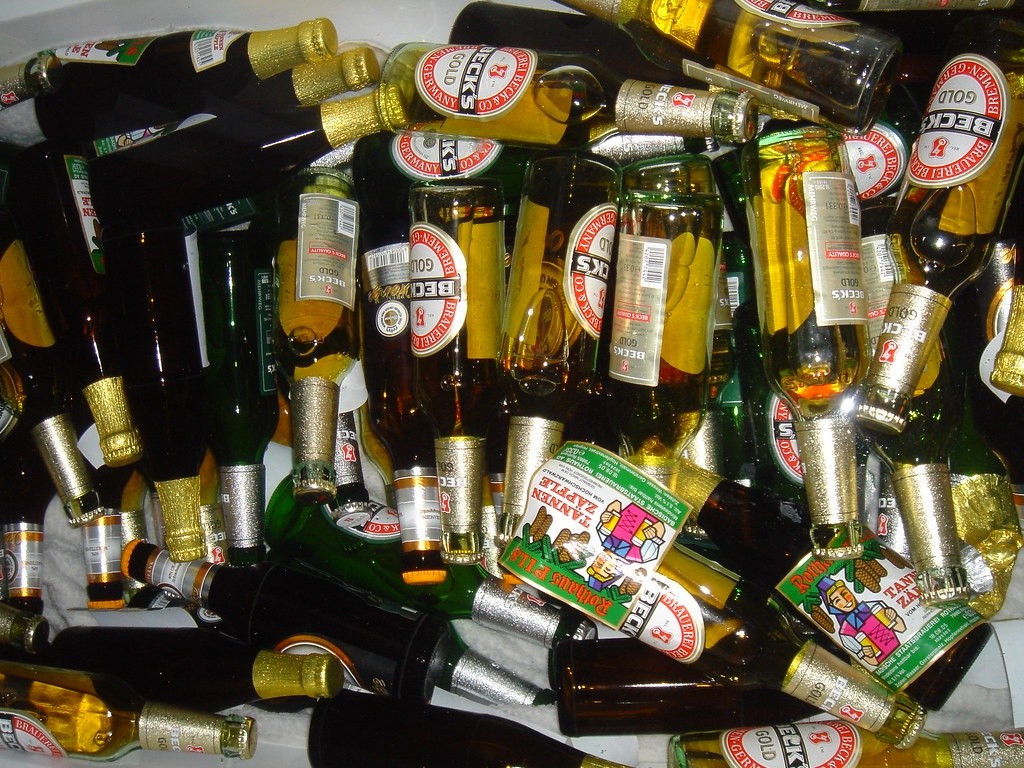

0,361,25,441
81,457,125,608
274,163,338,507
77,47,379,157
1,52,64,109
105,212,214,560
0,663,255,761
0,142,105,528
338,3,1024,768
86,86,405,228
120,535,453,703
0,429,55,614
115,469,153,605
200,222,283,564
0,603,49,657
196,448,229,566
34,14,338,139
13,140,142,467
266,467,596,647
39,627,346,715
